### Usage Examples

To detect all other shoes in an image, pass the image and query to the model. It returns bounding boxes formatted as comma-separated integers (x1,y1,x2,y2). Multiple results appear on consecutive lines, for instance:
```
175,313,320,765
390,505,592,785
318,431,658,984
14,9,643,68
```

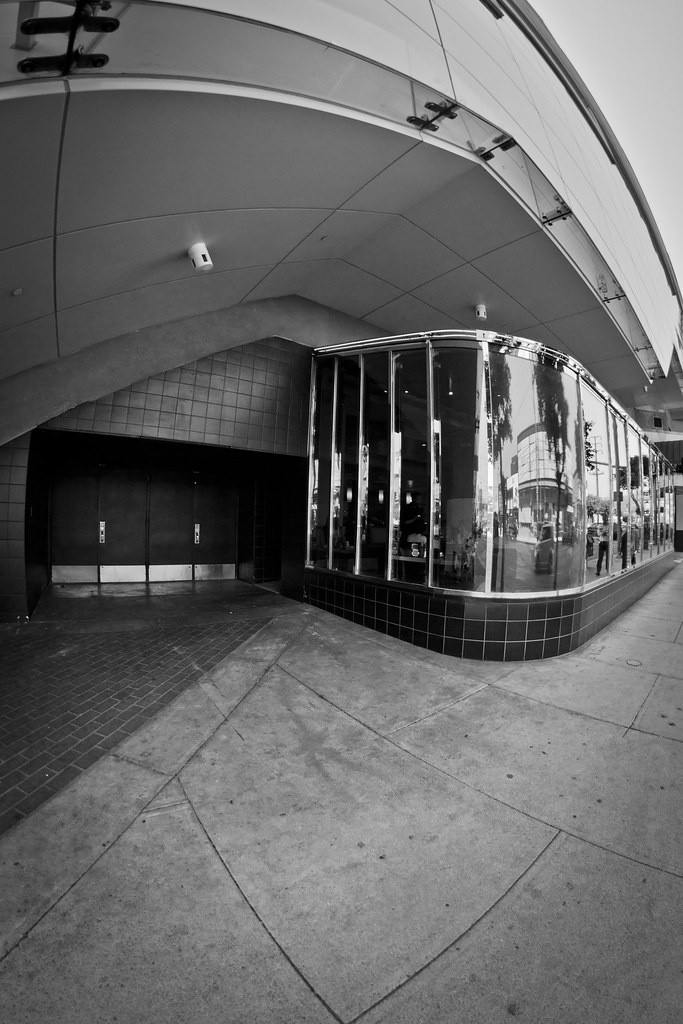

595,573,600,575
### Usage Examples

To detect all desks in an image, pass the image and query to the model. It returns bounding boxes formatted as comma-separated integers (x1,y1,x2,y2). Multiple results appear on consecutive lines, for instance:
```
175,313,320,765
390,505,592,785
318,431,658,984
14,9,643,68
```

382,549,472,587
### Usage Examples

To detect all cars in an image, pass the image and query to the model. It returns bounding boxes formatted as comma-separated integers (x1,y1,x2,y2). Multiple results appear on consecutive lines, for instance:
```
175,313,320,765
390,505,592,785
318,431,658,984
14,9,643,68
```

533,521,668,575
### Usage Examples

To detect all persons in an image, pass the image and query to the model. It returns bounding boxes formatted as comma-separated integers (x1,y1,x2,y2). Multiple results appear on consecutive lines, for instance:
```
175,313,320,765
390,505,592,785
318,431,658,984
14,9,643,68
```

398,491,427,546
619,527,639,570
594,511,609,575
506,509,518,542
